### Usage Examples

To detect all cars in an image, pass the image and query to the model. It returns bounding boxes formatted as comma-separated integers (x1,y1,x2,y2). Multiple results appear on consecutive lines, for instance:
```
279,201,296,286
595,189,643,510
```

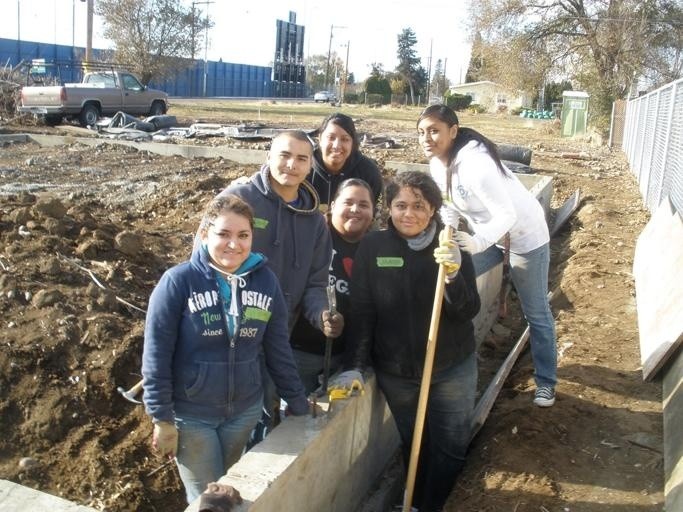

314,91,337,103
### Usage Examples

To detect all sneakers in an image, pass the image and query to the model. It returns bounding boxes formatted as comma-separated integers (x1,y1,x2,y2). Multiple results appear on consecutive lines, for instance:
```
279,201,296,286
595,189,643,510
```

533,384,558,409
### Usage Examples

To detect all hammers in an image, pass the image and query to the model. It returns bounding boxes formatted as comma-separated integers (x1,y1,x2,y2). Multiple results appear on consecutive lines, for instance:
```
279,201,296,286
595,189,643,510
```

117,380,144,405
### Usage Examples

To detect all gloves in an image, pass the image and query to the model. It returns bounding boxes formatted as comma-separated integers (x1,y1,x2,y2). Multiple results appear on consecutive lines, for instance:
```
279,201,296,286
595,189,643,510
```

439,204,460,228
197,479,244,512
152,418,179,463
322,309,347,340
453,230,479,256
433,239,462,280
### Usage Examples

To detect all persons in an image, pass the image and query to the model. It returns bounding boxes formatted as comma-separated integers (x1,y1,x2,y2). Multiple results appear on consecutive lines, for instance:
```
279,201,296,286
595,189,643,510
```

346,170,481,510
306,114,382,206
188,131,344,460
141,193,321,503
417,104,557,407
289,177,373,396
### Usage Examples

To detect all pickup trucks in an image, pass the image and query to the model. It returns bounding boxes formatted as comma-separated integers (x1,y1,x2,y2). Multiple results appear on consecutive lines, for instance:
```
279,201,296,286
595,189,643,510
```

18,70,169,127
